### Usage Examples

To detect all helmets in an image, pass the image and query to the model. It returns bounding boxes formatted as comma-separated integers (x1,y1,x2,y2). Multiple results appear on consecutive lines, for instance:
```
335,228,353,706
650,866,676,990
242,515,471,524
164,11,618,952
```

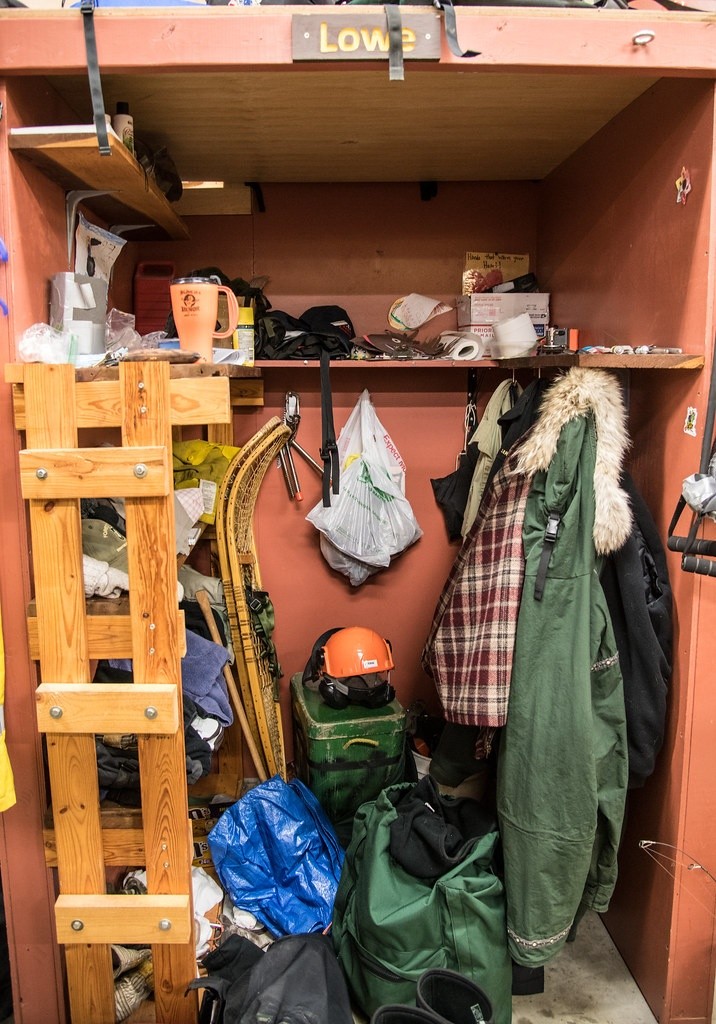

316,623,394,677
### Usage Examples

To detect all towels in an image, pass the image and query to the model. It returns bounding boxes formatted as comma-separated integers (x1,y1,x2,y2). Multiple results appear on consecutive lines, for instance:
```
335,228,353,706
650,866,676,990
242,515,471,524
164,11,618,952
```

108,627,234,728
82,552,185,603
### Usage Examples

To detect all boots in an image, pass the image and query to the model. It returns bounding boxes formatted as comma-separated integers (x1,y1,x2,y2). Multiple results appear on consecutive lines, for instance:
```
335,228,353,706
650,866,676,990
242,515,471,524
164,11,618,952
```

371,968,494,1024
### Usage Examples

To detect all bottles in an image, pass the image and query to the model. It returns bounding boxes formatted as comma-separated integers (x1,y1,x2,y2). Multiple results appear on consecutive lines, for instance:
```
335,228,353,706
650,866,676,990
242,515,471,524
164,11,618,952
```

113,102,133,155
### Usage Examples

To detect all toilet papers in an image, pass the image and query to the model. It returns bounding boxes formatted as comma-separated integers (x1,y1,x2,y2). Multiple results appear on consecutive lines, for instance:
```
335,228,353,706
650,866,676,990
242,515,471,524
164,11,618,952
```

449,338,479,361
492,311,539,357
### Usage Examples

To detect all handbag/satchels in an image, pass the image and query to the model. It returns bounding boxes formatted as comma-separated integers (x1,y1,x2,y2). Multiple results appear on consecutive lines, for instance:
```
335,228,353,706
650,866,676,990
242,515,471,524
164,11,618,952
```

303,388,425,587
429,367,481,543
207,774,346,938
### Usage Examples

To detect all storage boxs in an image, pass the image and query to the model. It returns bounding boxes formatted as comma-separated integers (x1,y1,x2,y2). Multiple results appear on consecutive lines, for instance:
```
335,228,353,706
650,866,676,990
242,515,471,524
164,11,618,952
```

455,293,551,328
457,323,546,357
291,671,408,824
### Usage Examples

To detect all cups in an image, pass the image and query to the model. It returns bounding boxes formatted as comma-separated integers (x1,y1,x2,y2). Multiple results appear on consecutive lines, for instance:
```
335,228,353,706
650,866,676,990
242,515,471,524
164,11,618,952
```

170,277,238,363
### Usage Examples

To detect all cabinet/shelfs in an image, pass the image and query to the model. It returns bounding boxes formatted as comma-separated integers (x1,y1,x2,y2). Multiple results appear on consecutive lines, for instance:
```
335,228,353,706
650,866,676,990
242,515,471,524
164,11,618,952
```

7,361,248,1024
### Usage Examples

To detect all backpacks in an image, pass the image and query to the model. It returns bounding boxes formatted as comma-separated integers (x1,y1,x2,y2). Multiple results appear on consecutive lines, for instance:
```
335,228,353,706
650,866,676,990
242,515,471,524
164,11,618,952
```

183,928,354,1024
331,772,512,1024
